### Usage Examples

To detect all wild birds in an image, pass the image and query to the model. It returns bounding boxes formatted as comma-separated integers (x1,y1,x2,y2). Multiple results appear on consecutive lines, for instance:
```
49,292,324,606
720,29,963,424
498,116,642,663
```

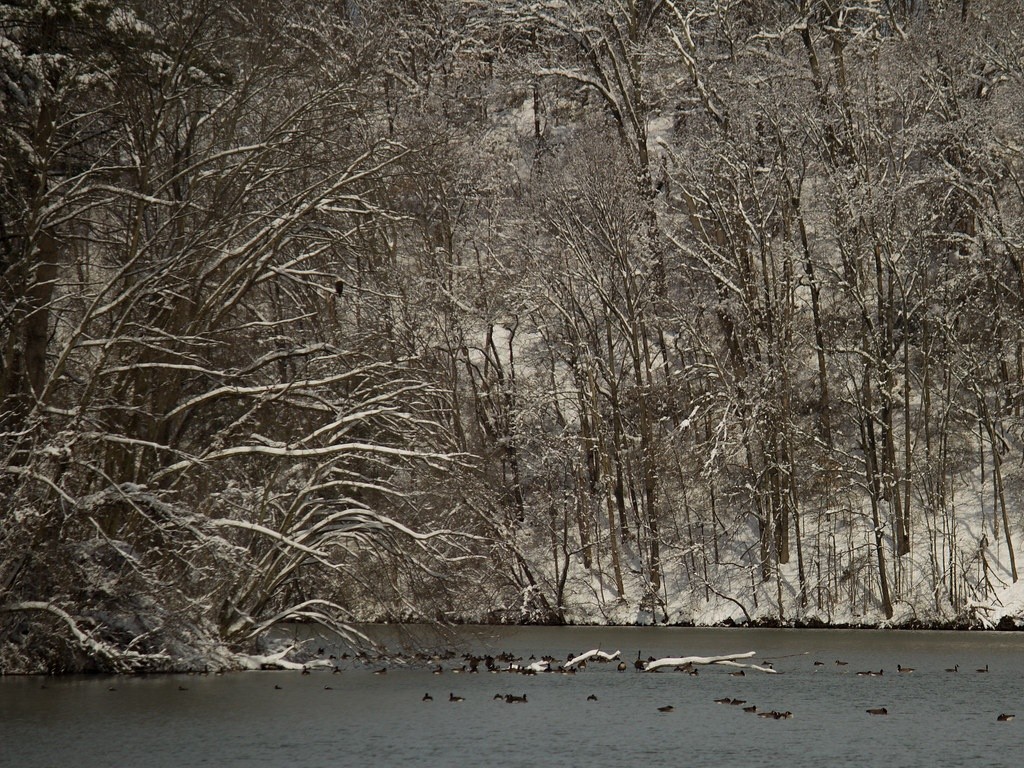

106,634,1017,722
